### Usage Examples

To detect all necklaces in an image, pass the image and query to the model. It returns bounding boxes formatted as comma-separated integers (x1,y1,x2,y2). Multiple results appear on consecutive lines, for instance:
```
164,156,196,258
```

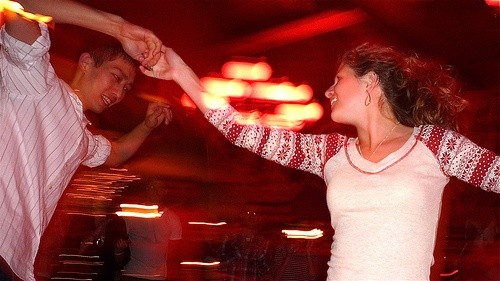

357,122,401,160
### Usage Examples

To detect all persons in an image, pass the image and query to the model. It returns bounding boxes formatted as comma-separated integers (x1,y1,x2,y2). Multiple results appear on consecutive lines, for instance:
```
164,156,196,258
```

139,41,500,281
0,0,173,281
